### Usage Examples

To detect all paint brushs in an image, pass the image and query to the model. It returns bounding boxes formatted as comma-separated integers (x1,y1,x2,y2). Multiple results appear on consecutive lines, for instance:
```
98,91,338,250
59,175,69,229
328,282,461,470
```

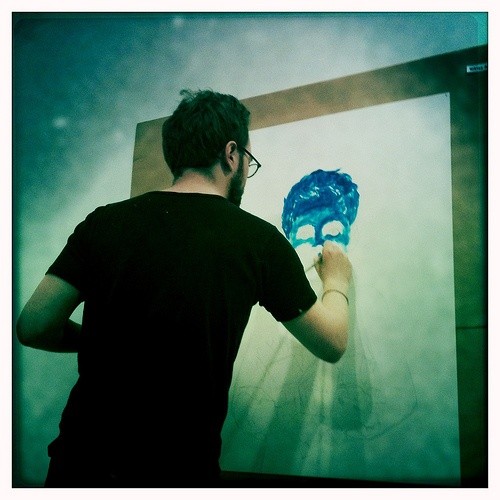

304,252,323,275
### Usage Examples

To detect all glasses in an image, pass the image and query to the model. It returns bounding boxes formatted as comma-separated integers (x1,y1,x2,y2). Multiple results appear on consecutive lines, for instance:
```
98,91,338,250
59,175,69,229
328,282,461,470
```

234,139,261,178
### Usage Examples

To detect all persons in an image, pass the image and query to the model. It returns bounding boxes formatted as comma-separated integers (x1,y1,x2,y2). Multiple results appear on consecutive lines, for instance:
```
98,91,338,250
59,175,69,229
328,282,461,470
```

14,89,353,488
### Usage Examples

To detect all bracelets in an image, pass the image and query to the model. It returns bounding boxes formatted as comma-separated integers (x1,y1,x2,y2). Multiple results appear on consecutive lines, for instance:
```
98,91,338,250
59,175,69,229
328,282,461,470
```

321,289,349,305
